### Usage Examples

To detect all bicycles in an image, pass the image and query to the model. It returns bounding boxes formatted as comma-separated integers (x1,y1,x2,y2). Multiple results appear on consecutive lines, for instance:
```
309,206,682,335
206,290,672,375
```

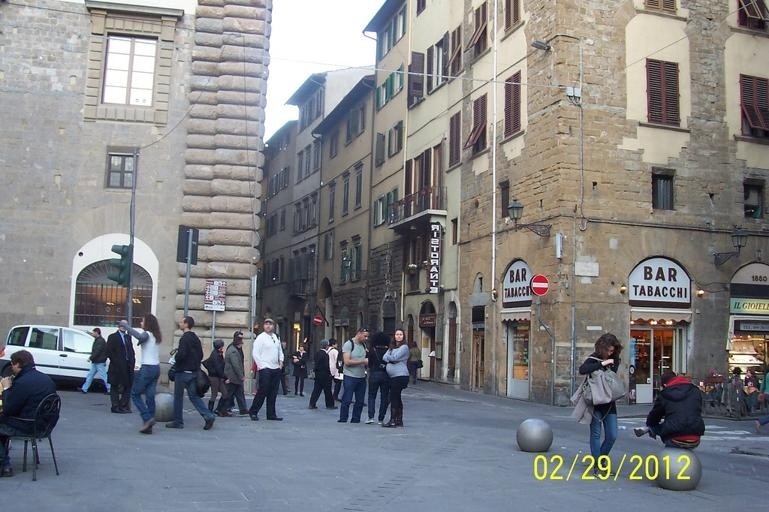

730,376,748,421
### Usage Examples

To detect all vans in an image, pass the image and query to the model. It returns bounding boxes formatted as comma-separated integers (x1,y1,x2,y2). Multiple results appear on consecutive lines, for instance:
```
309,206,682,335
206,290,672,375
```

0,323,110,388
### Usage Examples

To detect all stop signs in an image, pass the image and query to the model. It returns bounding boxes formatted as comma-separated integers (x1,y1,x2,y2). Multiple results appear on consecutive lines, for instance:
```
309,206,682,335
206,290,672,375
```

530,275,550,296
313,314,323,326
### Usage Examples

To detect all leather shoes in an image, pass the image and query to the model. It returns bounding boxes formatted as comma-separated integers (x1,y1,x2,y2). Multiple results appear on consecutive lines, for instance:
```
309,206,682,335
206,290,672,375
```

0,468,13,477
396,421,403,426
382,421,397,427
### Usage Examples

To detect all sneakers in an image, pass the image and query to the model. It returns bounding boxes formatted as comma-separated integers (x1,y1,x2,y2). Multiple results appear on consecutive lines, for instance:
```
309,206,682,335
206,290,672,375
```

633,427,649,436
754,420,761,433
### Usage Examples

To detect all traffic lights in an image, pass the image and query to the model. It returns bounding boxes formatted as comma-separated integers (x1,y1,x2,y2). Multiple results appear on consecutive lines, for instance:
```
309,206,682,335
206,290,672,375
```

106,244,132,287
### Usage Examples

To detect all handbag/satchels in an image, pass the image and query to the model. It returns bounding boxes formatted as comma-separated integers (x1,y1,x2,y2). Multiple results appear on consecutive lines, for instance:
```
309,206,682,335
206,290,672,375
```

417,360,423,368
169,364,176,381
584,369,626,405
197,367,211,398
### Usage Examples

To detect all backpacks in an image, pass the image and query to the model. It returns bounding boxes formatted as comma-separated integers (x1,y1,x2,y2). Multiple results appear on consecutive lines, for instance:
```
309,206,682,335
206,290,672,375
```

372,345,388,370
336,339,365,373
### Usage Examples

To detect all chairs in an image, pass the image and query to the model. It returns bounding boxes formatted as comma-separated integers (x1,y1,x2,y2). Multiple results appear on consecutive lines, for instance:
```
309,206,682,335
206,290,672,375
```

0,394,61,481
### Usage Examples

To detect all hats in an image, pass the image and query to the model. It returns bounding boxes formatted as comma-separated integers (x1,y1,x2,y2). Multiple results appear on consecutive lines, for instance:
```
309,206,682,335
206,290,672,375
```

731,367,742,373
214,340,223,348
234,337,242,345
264,318,274,323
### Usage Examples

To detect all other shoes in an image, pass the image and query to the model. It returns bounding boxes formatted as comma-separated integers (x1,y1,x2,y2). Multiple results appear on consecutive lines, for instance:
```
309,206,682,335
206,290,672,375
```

77,387,87,394
203,415,215,430
144,426,152,434
224,410,233,416
104,392,111,395
309,405,318,409
111,407,126,414
267,416,283,421
249,411,258,421
350,419,361,423
377,420,383,425
166,423,184,429
119,408,132,413
337,420,347,422
214,408,225,417
295,391,298,395
365,418,374,423
139,418,155,432
326,406,338,409
240,410,249,414
282,391,287,395
300,392,304,396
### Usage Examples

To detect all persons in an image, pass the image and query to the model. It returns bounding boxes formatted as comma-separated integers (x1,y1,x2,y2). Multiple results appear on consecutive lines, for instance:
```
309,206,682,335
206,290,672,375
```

633,371,703,450
1,350,56,479
205,319,421,427
1,343,17,448
728,366,768,434
76,328,111,396
579,333,622,479
104,319,136,415
165,316,215,430
116,315,161,434
